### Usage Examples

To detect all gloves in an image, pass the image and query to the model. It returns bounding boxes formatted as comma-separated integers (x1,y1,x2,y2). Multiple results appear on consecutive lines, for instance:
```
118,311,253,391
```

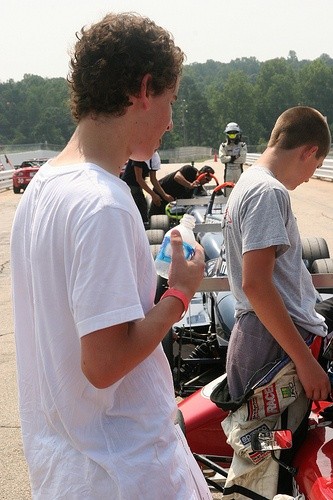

229,155,236,164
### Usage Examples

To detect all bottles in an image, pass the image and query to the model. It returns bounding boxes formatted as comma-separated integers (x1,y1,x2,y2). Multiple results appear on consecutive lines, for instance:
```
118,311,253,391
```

155,214,197,278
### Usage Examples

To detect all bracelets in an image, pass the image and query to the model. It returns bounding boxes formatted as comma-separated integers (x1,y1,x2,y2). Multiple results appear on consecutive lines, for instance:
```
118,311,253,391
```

160,289,189,322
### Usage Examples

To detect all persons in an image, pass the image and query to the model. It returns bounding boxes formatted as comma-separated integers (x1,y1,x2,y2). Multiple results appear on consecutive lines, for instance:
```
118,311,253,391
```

219,122,247,197
210,105,333,500
122,151,215,229
9,10,213,500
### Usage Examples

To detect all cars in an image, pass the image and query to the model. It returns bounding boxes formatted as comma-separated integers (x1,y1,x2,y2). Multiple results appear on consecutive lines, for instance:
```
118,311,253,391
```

12,159,50,194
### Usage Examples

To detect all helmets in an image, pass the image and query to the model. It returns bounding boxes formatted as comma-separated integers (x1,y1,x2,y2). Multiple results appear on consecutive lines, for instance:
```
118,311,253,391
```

224,122,241,133
